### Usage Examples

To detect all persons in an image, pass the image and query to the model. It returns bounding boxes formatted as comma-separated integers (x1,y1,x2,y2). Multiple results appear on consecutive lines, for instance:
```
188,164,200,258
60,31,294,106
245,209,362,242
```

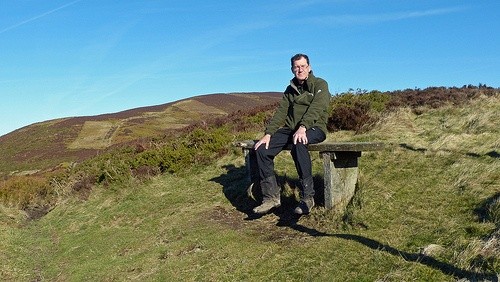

253,53,329,215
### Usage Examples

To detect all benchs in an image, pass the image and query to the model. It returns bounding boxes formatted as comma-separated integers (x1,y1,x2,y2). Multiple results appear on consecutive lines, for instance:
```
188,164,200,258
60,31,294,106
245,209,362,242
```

231,139,385,212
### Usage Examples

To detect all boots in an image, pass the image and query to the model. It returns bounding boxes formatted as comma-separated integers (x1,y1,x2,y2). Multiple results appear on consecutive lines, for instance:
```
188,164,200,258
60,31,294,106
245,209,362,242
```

253,175,281,213
294,176,315,214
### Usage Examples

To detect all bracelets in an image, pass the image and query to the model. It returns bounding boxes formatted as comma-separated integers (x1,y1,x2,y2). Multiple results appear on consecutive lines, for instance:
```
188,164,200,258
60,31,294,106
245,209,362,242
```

301,125,305,127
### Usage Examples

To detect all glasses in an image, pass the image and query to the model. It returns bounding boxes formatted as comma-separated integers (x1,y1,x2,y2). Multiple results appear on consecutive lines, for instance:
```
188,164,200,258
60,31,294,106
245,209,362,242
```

292,64,308,71
